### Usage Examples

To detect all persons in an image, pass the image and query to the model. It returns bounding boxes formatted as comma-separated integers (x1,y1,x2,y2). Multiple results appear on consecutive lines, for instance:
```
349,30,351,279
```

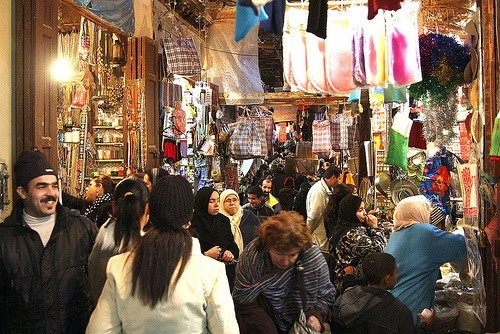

260,178,281,215
330,252,436,334
219,189,262,257
328,195,388,299
85,175,239,334
87,178,150,307
383,194,467,331
242,186,275,224
143,167,170,234
277,176,300,212
188,186,239,296
62,174,115,229
305,166,359,266
232,210,335,334
293,182,311,220
0,151,100,334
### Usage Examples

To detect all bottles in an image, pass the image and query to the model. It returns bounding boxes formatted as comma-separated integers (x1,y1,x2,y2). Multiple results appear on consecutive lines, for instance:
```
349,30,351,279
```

54,104,125,178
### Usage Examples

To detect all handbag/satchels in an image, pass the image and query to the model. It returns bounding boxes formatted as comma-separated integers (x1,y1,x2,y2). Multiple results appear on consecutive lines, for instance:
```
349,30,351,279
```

164,33,202,74
290,321,331,334
312,100,354,153
224,107,274,160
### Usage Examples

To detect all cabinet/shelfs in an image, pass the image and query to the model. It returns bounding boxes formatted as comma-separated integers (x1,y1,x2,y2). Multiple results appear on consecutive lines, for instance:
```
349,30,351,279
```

91,92,125,184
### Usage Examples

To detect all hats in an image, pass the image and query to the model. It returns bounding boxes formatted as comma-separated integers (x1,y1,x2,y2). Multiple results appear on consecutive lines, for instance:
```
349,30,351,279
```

149,175,194,229
284,177,295,188
15,150,58,187
300,182,312,192
